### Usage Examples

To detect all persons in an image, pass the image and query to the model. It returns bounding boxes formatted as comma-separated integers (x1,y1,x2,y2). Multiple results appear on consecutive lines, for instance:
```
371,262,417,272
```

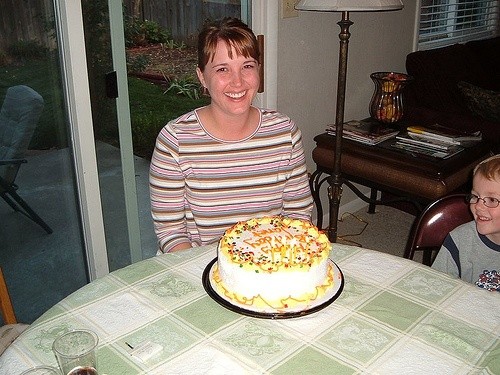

148,17,314,255
431,154,500,293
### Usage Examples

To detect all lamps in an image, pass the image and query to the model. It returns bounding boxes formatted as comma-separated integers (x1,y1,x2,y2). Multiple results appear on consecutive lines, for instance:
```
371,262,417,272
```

367,69,410,125
292,0,405,244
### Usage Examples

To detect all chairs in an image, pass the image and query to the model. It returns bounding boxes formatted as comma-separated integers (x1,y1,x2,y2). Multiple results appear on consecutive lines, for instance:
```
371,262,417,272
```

404,194,475,267
0,85,53,236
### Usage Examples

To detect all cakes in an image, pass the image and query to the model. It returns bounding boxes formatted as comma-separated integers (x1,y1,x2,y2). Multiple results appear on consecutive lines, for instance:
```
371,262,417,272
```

212,216,332,308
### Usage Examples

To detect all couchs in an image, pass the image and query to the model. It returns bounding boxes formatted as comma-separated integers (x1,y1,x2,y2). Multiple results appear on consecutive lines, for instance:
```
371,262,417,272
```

401,36,500,158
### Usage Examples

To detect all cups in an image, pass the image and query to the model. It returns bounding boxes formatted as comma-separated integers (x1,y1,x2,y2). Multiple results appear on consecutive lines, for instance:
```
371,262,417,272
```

52,328,99,375
19,365,62,375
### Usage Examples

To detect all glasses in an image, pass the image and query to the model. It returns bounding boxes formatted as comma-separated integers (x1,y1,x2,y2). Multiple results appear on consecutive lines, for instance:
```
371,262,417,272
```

465,194,500,208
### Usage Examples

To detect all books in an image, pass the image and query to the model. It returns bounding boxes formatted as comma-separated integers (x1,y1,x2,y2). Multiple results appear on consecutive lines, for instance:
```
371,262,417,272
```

326,120,482,159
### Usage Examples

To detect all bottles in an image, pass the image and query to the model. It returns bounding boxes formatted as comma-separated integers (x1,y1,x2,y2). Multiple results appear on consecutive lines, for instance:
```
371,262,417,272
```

369,70,407,123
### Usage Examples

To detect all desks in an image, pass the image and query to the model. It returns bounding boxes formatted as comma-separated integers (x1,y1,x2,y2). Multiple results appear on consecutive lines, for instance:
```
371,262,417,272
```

305,117,491,232
0,244,500,375
